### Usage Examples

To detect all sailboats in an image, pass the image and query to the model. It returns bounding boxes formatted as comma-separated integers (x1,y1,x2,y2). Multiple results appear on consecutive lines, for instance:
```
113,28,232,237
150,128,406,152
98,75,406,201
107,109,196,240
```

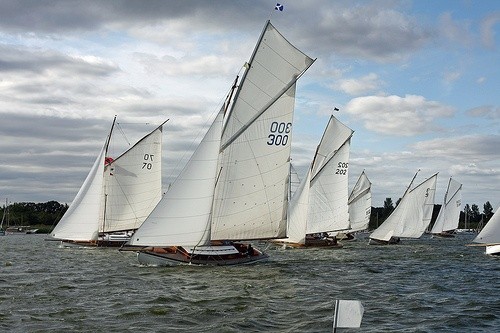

43,14,500,270
0,196,40,236
